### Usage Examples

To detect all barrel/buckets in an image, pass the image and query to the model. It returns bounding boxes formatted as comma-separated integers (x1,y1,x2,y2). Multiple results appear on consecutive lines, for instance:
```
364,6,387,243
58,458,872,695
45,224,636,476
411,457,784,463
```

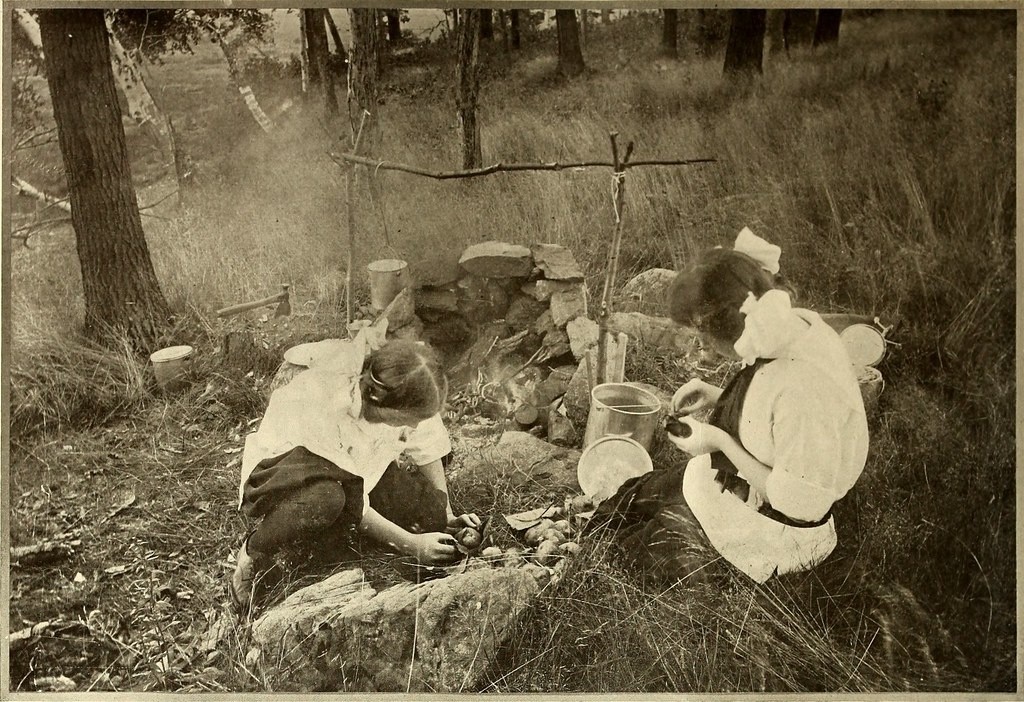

150,345,193,388
367,258,410,312
581,383,662,454
852,366,885,419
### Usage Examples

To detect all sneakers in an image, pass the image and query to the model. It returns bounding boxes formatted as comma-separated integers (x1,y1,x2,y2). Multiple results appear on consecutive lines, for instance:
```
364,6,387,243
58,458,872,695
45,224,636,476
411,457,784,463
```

231,529,262,612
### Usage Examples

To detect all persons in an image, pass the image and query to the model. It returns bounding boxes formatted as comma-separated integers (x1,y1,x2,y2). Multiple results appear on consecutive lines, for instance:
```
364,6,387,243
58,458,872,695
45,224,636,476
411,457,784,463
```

233,338,479,607
581,250,869,590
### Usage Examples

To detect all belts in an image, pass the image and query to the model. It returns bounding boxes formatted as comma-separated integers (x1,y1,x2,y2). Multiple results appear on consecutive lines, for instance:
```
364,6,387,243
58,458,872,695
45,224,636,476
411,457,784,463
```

729,479,831,528
